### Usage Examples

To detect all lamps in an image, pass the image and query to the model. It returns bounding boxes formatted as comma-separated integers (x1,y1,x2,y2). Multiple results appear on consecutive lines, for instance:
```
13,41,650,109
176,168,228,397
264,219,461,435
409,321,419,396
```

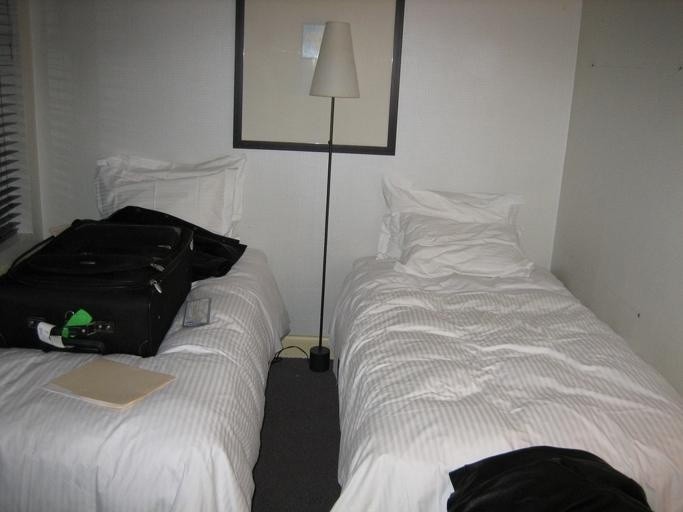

305,20,360,374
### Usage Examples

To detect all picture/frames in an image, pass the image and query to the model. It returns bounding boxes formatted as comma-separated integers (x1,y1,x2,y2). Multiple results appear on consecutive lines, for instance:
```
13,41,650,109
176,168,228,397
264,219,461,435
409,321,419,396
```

231,0,405,158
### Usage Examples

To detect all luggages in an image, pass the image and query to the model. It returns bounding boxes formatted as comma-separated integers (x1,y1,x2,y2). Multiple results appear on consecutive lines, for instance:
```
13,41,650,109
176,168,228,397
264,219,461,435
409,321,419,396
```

1,219,194,358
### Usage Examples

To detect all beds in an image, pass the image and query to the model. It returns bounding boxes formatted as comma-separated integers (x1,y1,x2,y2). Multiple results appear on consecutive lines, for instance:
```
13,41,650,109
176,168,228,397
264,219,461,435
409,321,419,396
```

0,245,291,512
328,253,682,511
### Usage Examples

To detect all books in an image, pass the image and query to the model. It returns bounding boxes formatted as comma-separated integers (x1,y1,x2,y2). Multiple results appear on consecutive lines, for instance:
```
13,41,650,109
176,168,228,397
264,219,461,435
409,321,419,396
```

41,354,176,412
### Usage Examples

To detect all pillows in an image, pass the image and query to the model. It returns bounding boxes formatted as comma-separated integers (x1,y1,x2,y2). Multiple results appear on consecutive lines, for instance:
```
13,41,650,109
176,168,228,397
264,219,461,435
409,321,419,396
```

373,177,532,277
92,151,248,243
